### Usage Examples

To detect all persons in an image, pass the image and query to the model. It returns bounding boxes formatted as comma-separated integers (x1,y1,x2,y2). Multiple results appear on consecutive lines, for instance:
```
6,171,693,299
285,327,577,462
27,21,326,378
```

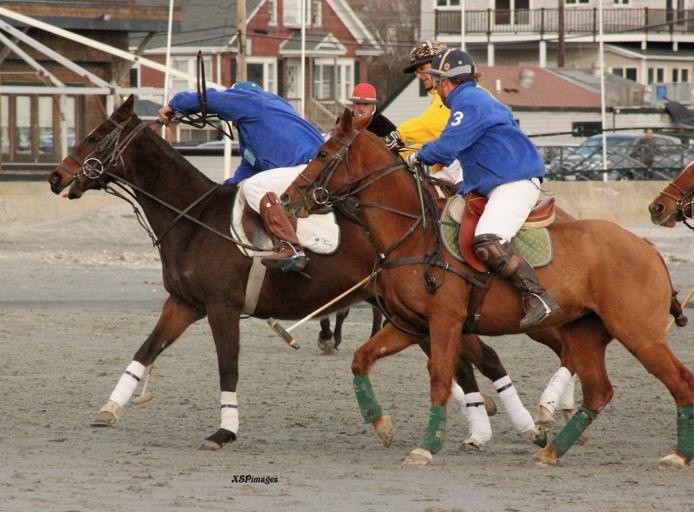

156,83,340,270
337,82,404,158
383,40,462,186
404,48,560,328
637,129,656,177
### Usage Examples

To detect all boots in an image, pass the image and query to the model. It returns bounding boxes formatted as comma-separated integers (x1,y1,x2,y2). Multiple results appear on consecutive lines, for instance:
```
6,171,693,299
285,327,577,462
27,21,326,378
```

258,190,308,272
469,232,559,329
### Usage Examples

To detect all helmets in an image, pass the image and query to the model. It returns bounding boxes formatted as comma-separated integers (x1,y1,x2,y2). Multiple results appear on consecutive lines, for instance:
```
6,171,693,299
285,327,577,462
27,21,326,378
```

228,81,262,93
347,83,377,105
419,49,474,80
402,42,447,74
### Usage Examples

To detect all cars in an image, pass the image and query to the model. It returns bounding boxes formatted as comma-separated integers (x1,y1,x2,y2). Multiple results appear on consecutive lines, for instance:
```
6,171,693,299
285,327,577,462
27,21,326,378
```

551,132,693,181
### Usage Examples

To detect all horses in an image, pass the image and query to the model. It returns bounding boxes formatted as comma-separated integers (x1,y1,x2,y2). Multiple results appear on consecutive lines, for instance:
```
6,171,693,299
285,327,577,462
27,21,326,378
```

47,93,547,452
334,113,591,452
649,159,694,228
319,304,383,355
278,107,694,472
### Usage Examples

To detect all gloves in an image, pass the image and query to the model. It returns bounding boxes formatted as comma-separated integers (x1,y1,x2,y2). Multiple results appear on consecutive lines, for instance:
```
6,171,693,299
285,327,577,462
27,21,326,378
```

383,131,399,150
404,153,418,165
